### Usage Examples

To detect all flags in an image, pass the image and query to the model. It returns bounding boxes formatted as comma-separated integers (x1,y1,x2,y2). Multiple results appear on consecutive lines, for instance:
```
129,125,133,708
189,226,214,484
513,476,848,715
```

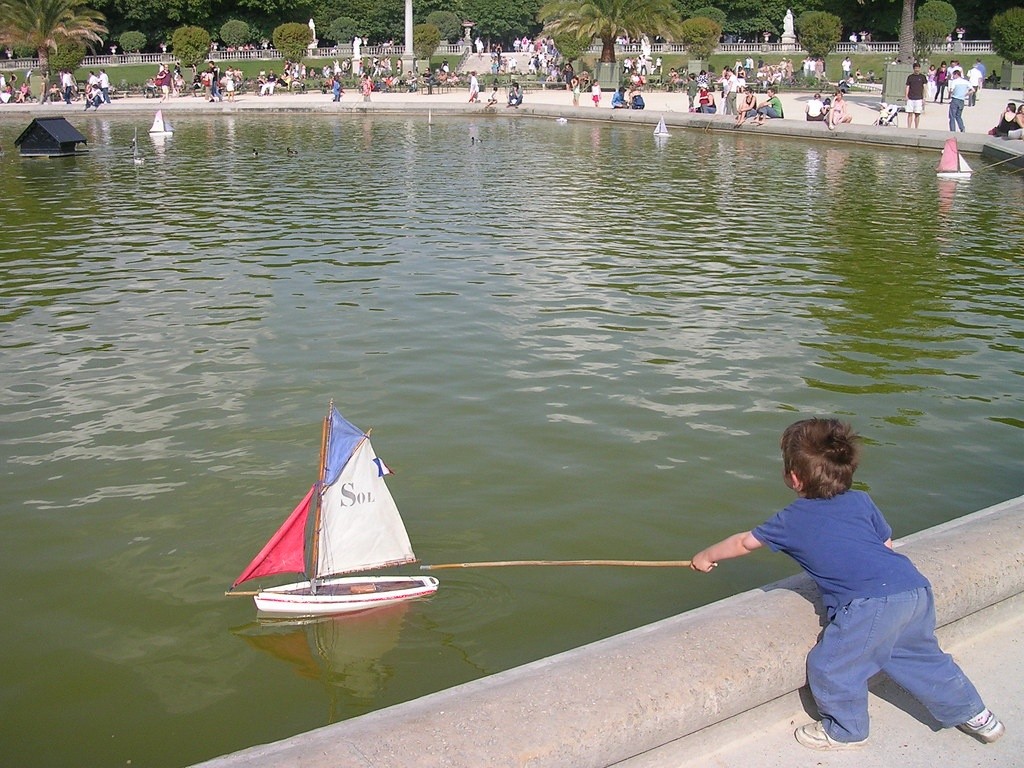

935,139,958,171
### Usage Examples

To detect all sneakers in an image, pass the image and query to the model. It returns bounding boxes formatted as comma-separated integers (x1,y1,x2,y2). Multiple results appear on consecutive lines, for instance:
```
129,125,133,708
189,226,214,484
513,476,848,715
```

956,707,1005,743
795,721,868,750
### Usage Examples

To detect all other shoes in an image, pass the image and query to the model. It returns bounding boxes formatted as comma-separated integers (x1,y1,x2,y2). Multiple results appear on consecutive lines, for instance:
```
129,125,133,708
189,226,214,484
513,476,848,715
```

828,123,835,129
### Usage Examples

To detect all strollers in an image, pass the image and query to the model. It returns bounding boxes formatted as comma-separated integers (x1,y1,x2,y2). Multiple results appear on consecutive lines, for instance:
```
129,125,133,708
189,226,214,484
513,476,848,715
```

869,98,904,127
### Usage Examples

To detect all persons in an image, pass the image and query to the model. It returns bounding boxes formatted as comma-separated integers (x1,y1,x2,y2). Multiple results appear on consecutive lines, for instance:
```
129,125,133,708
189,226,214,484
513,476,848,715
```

1007,104,1024,139
877,102,889,125
0,43,141,111
690,416,1005,748
591,79,601,107
251,146,299,156
994,103,1015,136
470,136,482,143
904,63,928,128
734,87,756,126
805,93,827,121
826,92,853,130
948,70,975,133
468,35,580,108
751,88,784,124
145,35,461,103
611,31,883,115
891,25,999,107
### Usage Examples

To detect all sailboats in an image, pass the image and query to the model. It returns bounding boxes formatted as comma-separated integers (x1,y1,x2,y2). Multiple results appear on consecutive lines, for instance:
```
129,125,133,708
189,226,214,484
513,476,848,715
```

128,127,145,167
650,114,673,138
209,404,441,618
936,136,976,178
145,101,174,135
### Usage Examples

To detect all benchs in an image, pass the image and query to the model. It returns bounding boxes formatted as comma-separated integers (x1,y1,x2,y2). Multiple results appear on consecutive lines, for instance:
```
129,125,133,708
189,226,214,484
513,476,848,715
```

78,77,776,100
